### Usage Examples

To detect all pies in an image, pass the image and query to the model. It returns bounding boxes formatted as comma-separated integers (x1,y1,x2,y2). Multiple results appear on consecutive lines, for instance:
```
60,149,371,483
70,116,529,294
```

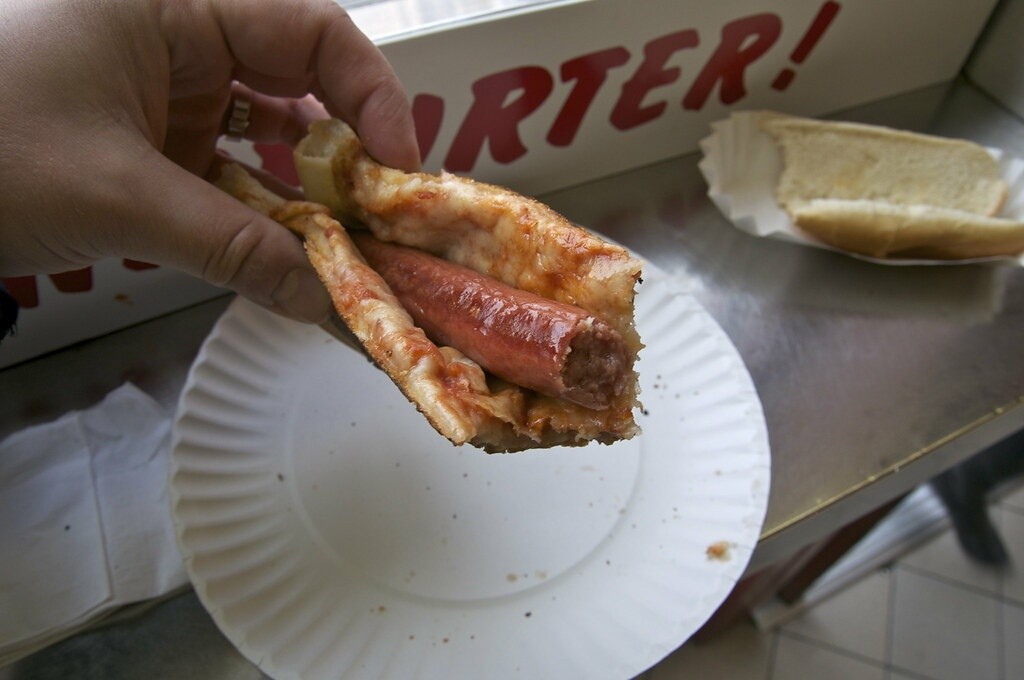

215,117,646,455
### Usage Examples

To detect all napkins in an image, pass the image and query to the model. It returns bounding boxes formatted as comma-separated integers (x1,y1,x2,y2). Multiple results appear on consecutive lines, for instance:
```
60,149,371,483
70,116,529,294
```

0,381,193,669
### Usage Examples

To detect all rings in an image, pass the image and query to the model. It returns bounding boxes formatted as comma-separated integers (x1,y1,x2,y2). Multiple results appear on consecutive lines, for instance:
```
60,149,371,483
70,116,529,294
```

225,96,250,137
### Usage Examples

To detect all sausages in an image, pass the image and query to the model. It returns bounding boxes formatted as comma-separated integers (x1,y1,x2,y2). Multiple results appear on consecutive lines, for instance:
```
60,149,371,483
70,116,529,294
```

339,223,629,412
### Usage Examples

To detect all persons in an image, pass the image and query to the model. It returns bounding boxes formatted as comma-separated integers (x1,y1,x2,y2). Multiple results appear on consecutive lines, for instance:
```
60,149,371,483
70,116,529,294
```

0,0,421,326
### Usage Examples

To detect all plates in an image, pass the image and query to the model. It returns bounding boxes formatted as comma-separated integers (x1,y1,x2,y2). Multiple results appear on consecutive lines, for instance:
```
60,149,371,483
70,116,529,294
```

698,110,1024,265
167,229,772,680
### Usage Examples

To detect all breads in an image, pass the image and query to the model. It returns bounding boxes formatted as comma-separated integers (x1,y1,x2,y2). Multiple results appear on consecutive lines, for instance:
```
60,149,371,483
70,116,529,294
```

762,117,1023,258
